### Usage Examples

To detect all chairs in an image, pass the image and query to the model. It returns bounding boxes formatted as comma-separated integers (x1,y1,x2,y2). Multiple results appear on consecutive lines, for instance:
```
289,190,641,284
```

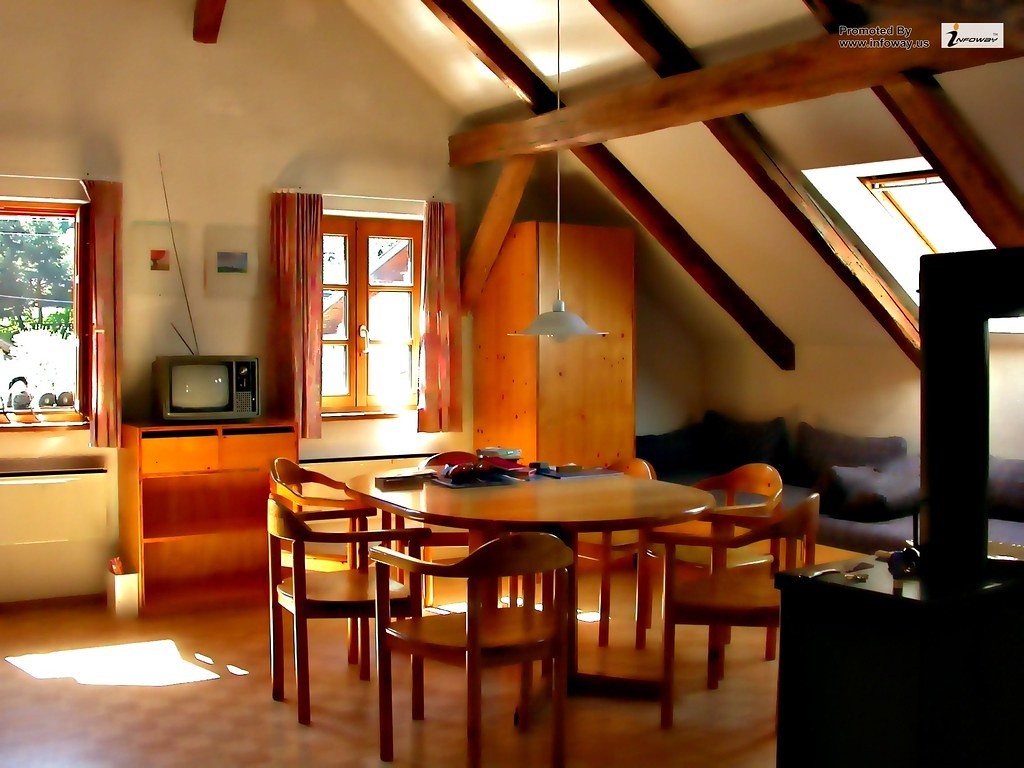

647,463,782,661
266,499,432,726
267,458,379,665
369,534,574,768
577,460,655,652
650,493,819,729
423,451,480,609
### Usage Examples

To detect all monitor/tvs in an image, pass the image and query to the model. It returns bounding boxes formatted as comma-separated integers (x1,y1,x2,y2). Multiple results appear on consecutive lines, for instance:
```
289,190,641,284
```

150,355,262,422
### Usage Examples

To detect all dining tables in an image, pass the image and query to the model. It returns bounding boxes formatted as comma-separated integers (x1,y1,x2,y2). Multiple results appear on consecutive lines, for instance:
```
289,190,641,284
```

345,459,714,732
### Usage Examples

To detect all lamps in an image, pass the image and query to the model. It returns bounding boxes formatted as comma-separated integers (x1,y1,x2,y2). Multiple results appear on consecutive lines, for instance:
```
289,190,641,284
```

507,0,610,337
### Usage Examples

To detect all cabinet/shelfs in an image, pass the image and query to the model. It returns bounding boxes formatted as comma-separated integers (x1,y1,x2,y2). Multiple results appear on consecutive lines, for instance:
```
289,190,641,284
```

472,222,631,572
117,417,300,616
776,246,1023,768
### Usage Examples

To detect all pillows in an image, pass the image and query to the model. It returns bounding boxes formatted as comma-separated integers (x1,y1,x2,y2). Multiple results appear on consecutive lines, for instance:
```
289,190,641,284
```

694,411,792,483
989,455,1024,521
634,424,700,476
788,423,906,488
812,455,921,521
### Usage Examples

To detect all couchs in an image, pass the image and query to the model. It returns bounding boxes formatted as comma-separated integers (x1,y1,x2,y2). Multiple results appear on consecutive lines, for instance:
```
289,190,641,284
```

660,474,1023,560
